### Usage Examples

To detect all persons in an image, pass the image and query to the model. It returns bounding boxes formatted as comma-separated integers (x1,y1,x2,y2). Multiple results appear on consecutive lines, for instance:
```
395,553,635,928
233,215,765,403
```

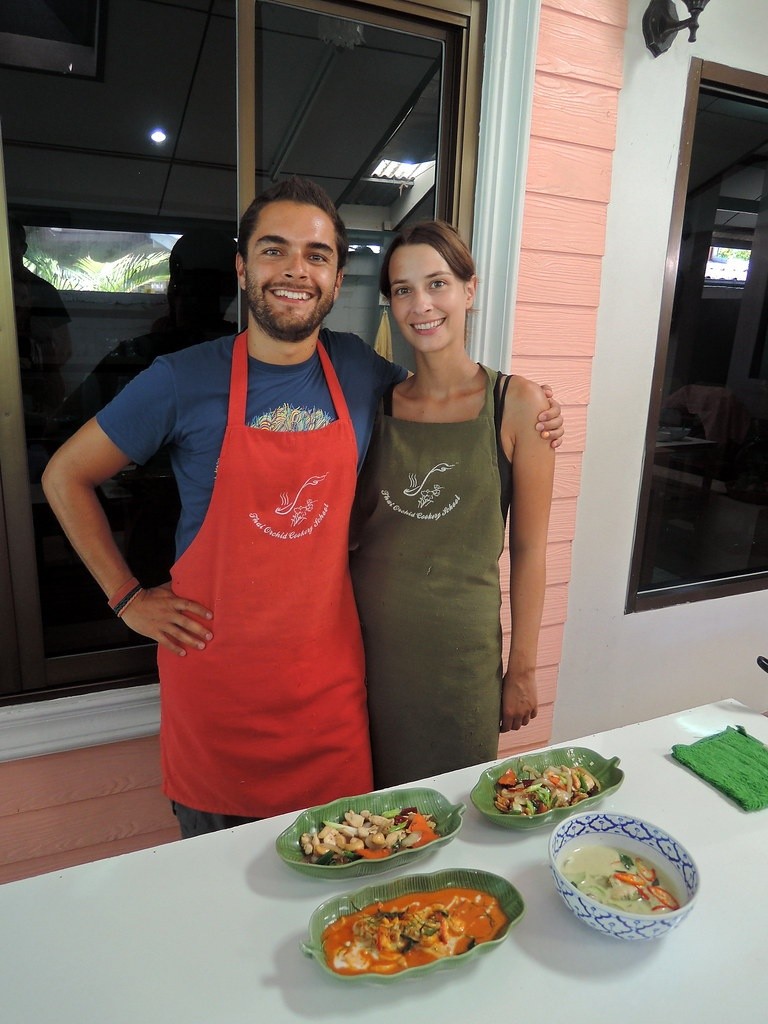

346,221,556,791
42,175,564,841
36,228,239,459
8,217,72,424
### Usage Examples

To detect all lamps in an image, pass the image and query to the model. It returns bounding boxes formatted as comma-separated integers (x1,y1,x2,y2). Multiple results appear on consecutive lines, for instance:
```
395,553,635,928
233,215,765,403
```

643,0,709,57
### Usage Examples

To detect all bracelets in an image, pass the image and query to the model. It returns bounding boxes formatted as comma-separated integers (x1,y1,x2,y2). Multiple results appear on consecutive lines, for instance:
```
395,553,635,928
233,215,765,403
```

108,577,143,617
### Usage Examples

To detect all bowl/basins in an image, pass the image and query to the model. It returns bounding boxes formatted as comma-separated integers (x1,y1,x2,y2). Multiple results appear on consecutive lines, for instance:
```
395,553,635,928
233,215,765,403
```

547,811,702,941
275,788,464,880
296,866,527,985
471,746,625,832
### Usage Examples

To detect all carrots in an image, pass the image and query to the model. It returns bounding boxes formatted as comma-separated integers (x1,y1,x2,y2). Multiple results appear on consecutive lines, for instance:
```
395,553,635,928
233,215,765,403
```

356,811,437,859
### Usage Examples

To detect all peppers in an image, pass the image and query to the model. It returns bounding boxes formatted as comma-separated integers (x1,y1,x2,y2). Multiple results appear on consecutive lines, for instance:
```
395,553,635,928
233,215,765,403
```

613,857,682,911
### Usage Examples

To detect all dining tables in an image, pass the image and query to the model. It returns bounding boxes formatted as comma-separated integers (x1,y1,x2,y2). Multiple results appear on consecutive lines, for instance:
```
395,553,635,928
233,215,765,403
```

638,426,718,590
0,696,768,1024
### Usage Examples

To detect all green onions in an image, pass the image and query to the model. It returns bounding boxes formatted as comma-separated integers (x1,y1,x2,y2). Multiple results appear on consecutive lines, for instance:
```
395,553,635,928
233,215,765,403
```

506,769,589,815
313,808,407,865
